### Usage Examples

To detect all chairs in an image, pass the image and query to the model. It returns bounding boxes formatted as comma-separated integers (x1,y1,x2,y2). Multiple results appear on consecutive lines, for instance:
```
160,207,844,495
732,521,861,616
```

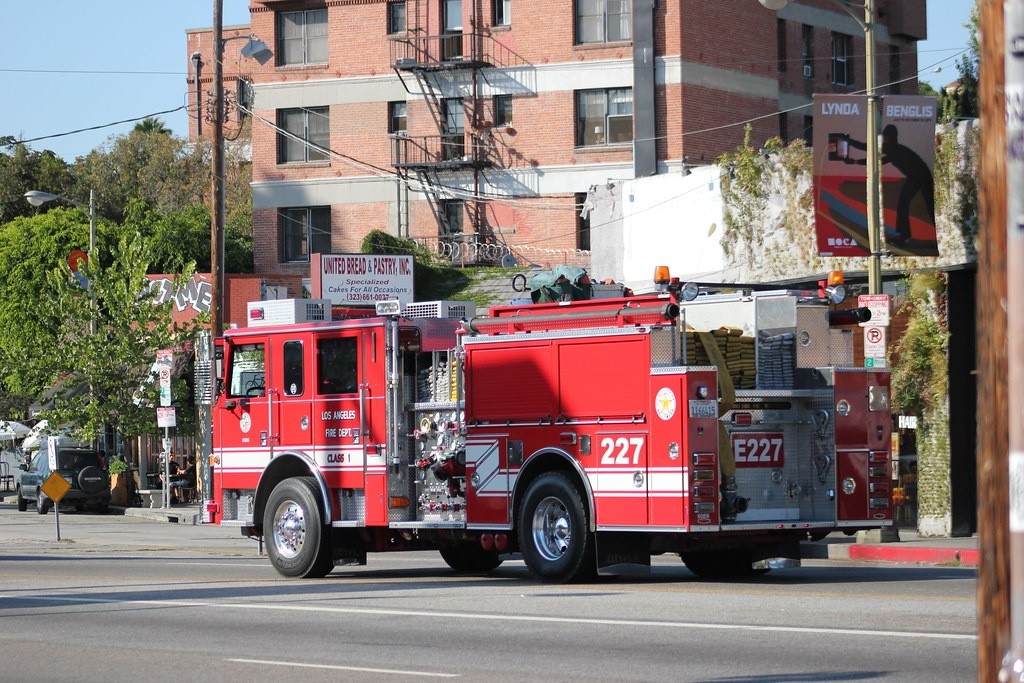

179,473,196,504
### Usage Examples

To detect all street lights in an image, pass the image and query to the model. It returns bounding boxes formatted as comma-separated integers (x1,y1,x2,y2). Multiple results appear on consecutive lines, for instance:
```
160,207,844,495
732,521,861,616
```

24,189,95,452
759,0,901,544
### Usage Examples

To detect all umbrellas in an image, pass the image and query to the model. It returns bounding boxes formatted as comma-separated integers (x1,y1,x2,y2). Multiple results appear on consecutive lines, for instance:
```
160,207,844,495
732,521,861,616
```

0,419,82,452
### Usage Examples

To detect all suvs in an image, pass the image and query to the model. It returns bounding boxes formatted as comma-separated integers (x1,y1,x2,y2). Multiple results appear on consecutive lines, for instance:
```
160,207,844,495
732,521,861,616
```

15,423,110,514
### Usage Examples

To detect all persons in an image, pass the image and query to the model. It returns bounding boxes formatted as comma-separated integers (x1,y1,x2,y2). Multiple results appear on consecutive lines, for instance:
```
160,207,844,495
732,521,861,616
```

158,450,197,505
96,449,108,471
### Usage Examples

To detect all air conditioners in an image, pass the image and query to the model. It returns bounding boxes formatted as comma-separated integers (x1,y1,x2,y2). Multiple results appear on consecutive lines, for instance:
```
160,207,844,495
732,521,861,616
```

803,65,811,76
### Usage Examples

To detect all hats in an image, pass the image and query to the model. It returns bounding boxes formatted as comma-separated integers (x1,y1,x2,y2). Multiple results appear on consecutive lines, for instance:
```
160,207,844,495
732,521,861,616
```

98,450,106,457
185,455,195,464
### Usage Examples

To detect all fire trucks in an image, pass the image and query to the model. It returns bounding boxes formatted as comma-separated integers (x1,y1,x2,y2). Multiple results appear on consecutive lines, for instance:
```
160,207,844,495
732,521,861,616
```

199,266,895,586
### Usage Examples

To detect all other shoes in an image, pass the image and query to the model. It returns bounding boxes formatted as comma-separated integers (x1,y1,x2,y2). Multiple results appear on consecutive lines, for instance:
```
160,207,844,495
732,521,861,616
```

170,496,181,503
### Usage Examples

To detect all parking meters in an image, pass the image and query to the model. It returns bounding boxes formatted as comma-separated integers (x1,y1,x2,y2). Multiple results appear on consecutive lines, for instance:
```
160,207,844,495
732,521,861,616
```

159,452,166,508
